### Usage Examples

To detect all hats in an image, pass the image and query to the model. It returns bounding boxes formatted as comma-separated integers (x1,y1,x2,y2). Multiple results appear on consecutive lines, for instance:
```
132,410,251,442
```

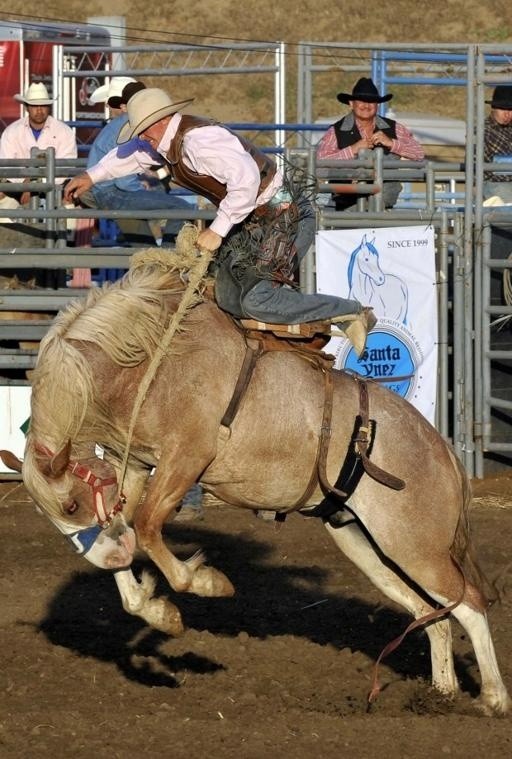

115,87,195,146
109,81,147,109
14,82,60,107
336,78,393,104
484,83,511,112
90,76,136,103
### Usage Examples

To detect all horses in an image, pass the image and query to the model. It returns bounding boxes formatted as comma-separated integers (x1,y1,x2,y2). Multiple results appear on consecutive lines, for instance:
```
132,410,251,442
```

2,262,511,721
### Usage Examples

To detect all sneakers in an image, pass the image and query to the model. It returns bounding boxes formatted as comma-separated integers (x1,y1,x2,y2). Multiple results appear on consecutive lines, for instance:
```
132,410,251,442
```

344,306,370,359
171,504,204,524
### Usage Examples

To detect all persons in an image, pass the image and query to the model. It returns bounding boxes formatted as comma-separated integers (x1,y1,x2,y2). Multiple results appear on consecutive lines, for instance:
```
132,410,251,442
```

90,74,136,120
315,76,424,212
77,78,194,209
0,81,82,223
465,84,512,204
62,87,378,359
